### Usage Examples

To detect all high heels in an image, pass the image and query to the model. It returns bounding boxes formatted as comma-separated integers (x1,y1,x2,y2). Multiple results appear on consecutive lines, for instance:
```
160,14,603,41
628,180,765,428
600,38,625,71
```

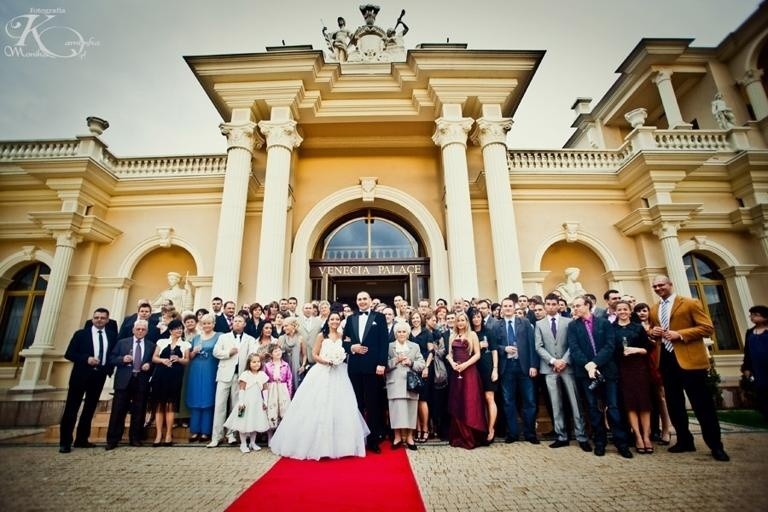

658,433,671,445
634,441,646,454
419,431,430,443
414,431,422,442
645,447,653,453
163,434,173,447
487,432,495,444
152,433,163,447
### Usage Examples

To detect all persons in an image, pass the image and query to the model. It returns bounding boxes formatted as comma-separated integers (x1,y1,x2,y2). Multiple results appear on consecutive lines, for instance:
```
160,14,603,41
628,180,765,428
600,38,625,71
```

384,8,410,63
552,265,584,307
738,304,768,428
711,89,738,129
648,273,731,462
85,291,671,459
60,307,117,454
147,270,193,317
315,15,352,68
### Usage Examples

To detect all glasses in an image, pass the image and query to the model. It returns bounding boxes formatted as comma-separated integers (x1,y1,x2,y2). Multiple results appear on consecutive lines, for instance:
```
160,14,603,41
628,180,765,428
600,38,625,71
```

652,284,665,288
94,316,105,320
139,310,150,313
572,304,581,308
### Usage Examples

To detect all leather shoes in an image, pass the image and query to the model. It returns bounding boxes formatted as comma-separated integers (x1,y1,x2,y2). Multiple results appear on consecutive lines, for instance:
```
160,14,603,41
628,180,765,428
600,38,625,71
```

129,441,142,447
189,434,199,442
59,445,70,453
405,440,417,450
525,436,540,444
667,442,696,453
225,432,237,444
594,443,607,456
505,436,519,443
549,439,569,448
239,444,250,454
618,447,632,458
199,435,210,442
206,440,219,447
578,441,592,452
106,443,118,450
392,438,403,448
711,447,730,461
249,443,261,451
73,442,96,448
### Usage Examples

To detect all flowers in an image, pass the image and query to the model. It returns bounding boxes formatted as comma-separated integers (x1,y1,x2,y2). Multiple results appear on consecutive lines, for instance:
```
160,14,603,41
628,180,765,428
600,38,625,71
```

323,344,347,369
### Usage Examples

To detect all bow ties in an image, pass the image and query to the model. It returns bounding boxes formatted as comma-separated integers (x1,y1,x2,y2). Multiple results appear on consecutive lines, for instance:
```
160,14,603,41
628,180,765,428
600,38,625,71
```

357,311,368,315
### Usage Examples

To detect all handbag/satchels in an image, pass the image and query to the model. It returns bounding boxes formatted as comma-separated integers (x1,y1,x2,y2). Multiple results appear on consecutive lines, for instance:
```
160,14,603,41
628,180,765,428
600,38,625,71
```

433,347,448,384
407,361,424,394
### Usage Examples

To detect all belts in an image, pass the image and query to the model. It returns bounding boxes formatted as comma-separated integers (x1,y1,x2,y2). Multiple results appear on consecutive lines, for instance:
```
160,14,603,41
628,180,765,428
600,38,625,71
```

508,357,519,362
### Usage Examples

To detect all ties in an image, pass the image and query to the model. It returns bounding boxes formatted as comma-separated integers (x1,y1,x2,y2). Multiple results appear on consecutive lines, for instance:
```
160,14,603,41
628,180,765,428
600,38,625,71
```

584,320,597,357
228,318,231,326
551,318,556,338
133,339,141,371
235,333,241,366
508,320,514,346
661,299,674,353
97,329,103,372
